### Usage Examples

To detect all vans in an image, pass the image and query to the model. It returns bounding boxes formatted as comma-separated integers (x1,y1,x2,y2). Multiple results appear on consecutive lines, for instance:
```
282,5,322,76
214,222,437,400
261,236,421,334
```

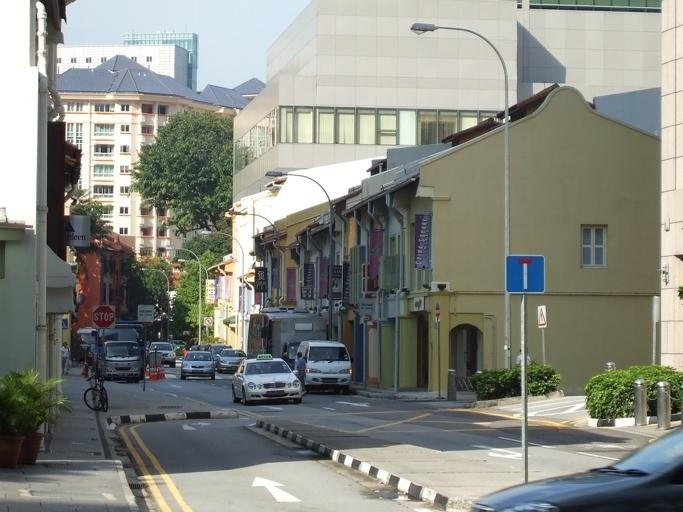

294,340,353,394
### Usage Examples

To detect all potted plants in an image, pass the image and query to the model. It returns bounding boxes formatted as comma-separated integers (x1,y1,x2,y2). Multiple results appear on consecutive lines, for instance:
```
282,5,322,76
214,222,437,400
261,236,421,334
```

0,368,74,469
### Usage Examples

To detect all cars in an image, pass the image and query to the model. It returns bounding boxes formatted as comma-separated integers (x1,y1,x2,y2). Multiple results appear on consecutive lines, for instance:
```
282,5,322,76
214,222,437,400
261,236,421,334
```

472,426,682,509
232,358,303,405
179,350,218,379
191,345,247,374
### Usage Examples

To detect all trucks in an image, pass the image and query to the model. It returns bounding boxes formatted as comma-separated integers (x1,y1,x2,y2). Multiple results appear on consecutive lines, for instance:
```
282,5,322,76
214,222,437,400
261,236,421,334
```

77,321,146,383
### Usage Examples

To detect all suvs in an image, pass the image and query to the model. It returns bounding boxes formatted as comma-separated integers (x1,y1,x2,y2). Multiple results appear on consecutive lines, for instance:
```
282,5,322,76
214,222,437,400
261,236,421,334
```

146,342,176,368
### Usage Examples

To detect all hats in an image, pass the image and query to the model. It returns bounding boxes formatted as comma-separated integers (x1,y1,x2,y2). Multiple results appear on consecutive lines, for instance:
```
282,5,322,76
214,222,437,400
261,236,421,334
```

63,341,68,344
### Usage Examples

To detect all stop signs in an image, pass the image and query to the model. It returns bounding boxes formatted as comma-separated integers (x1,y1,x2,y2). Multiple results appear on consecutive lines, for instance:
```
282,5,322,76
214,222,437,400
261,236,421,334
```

92,305,116,329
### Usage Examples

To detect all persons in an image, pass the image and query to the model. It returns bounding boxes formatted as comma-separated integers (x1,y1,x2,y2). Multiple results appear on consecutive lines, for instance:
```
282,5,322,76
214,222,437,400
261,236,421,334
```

182,345,189,359
295,352,306,393
62,342,70,373
83,346,94,365
515,345,531,365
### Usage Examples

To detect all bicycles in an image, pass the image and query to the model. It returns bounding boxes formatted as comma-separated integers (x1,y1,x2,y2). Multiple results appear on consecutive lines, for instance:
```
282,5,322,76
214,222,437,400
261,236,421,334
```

84,363,109,412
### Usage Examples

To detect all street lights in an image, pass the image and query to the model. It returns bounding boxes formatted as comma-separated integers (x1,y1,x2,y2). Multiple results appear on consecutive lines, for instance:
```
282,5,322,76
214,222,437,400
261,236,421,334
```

228,210,280,308
264,170,333,342
200,230,245,351
139,247,210,344
410,22,511,373
